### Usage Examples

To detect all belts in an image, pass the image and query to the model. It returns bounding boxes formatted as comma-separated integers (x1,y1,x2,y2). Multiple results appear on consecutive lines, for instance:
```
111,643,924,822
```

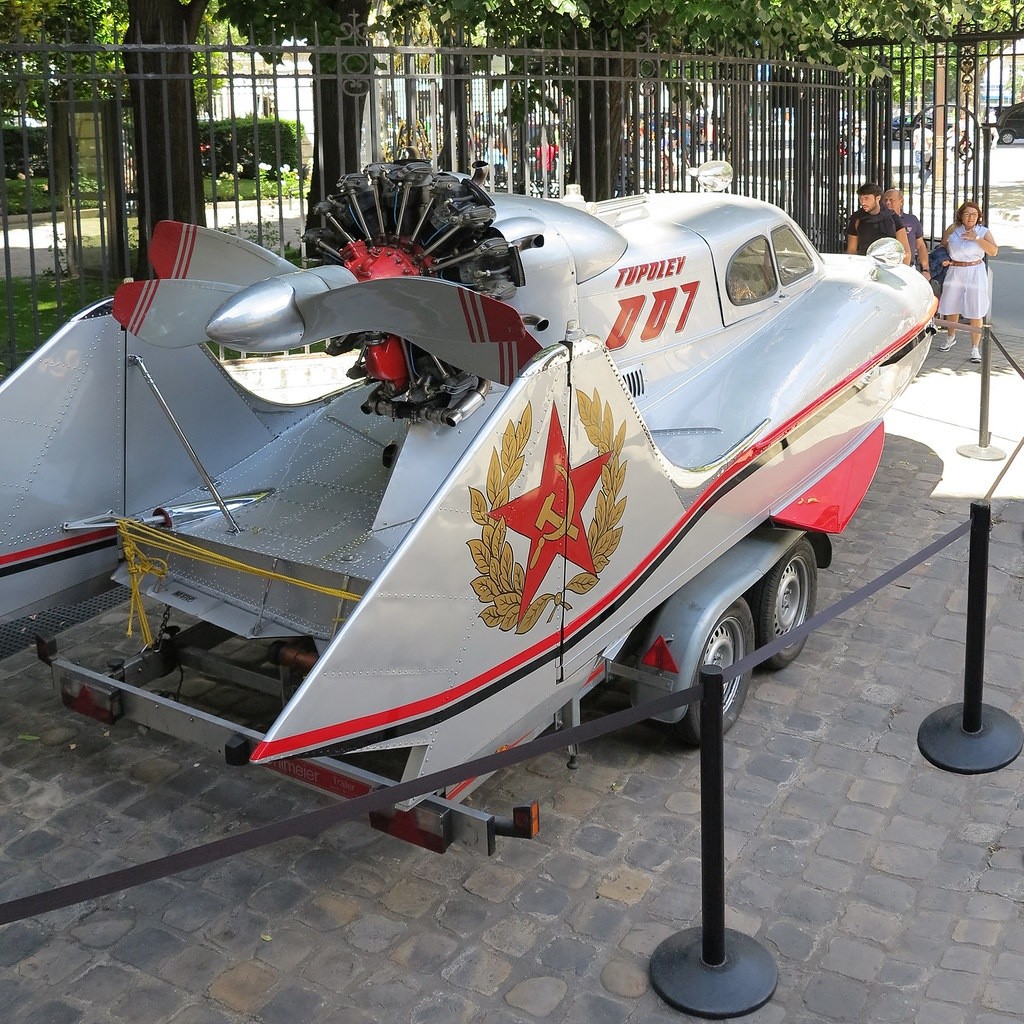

947,259,982,266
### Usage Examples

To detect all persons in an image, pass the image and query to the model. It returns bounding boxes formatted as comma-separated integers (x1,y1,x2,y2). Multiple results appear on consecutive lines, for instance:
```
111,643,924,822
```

883,189,931,281
846,183,912,265
482,123,569,183
911,106,999,196
639,119,695,174
938,202,999,362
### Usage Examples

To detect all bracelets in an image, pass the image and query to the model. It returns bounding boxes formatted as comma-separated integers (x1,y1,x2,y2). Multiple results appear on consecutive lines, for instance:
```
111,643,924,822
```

923,268,931,272
976,235,981,240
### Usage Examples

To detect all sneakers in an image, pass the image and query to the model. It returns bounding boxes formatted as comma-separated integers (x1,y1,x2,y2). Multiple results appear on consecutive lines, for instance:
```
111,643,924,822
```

939,335,956,352
970,348,982,363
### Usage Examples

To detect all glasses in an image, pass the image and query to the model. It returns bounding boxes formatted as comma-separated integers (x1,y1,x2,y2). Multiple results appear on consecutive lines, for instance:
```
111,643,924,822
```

962,211,980,217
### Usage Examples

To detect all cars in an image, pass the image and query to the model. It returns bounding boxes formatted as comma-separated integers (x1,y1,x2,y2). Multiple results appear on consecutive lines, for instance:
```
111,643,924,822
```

997,101,1024,143
882,115,953,142
